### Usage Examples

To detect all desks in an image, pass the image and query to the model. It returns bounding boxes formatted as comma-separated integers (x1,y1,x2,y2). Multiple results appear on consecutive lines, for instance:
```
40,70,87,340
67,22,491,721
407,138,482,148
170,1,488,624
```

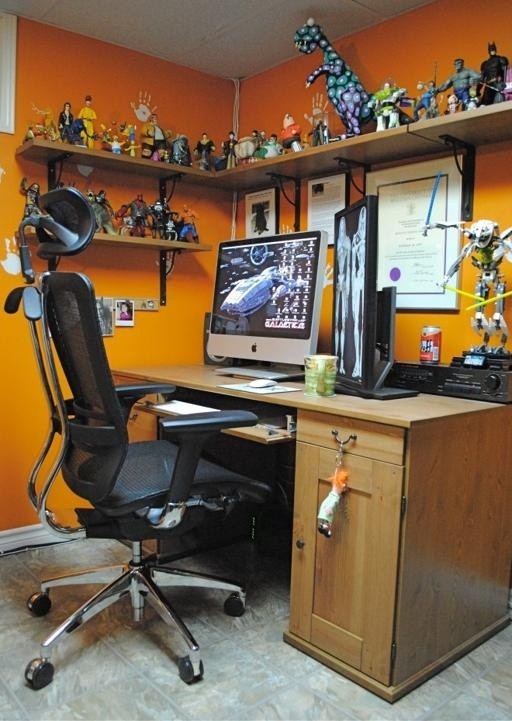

109,359,512,707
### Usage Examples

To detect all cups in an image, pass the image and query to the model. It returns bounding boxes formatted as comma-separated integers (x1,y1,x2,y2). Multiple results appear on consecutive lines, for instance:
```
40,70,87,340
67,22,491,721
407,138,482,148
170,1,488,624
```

301,355,340,398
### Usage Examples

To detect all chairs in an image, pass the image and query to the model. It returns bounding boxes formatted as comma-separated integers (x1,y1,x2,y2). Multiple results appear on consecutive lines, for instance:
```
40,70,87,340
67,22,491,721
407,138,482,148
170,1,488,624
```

11,183,271,698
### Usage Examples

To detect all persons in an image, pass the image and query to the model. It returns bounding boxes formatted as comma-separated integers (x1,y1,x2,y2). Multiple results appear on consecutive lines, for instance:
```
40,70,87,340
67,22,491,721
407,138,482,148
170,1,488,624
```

58,182,199,244
221,40,512,171
23,94,218,169
19,178,42,220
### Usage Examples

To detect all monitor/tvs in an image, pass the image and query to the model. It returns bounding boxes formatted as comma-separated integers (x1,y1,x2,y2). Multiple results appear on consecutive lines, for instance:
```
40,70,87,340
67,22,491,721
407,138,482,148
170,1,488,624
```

205,229,329,379
332,194,420,400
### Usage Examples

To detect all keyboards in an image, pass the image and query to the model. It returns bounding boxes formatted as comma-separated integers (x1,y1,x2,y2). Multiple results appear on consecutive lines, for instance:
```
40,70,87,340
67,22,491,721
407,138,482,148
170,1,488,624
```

151,399,222,417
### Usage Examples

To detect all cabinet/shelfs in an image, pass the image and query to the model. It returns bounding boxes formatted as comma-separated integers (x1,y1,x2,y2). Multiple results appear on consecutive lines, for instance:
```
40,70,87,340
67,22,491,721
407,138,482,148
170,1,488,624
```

14,135,214,305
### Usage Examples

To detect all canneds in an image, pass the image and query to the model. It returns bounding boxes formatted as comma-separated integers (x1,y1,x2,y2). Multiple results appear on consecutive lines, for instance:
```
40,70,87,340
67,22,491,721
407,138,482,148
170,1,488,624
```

419,325,441,364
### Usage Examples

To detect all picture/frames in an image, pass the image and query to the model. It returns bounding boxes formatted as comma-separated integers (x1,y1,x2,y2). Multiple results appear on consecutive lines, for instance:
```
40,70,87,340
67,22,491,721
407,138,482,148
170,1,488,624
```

363,152,463,314
305,171,352,250
242,185,279,238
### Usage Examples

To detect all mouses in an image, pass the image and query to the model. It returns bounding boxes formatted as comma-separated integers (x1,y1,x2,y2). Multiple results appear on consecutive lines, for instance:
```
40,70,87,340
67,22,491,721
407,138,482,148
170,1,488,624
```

249,379,277,388
